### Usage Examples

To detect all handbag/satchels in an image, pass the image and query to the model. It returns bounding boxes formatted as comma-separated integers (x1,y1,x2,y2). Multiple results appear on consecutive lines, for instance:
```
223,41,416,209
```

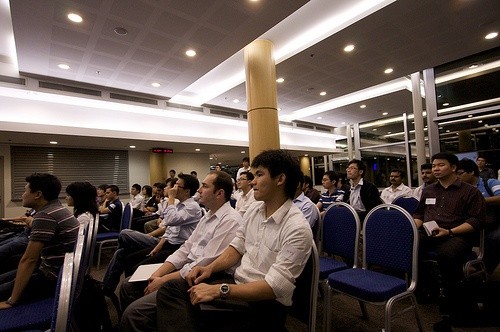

436,275,500,329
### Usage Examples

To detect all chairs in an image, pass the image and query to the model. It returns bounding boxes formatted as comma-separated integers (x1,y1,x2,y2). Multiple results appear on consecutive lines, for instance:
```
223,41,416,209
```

0,200,419,332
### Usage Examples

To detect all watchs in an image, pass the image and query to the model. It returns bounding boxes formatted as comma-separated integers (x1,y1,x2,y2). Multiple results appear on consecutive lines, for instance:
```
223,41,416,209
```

217,283,230,301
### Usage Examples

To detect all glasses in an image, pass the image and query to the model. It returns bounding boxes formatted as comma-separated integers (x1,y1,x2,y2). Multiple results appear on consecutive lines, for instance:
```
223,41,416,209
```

346,166,358,171
174,184,185,190
237,178,247,182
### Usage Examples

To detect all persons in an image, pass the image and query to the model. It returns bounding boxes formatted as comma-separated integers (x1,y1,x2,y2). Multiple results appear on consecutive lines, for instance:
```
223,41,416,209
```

0,182,97,262
96,184,122,234
413,150,486,332
340,160,381,231
315,170,346,219
0,173,80,309
215,157,320,231
121,149,312,332
380,157,500,236
103,170,202,292
113,170,244,317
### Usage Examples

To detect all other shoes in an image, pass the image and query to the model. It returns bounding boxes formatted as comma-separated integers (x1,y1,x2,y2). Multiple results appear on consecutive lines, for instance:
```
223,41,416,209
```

85,274,114,298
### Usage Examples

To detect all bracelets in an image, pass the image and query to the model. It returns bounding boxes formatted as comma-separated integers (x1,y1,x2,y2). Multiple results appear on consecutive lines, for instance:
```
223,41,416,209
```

6,297,17,307
448,229,454,237
